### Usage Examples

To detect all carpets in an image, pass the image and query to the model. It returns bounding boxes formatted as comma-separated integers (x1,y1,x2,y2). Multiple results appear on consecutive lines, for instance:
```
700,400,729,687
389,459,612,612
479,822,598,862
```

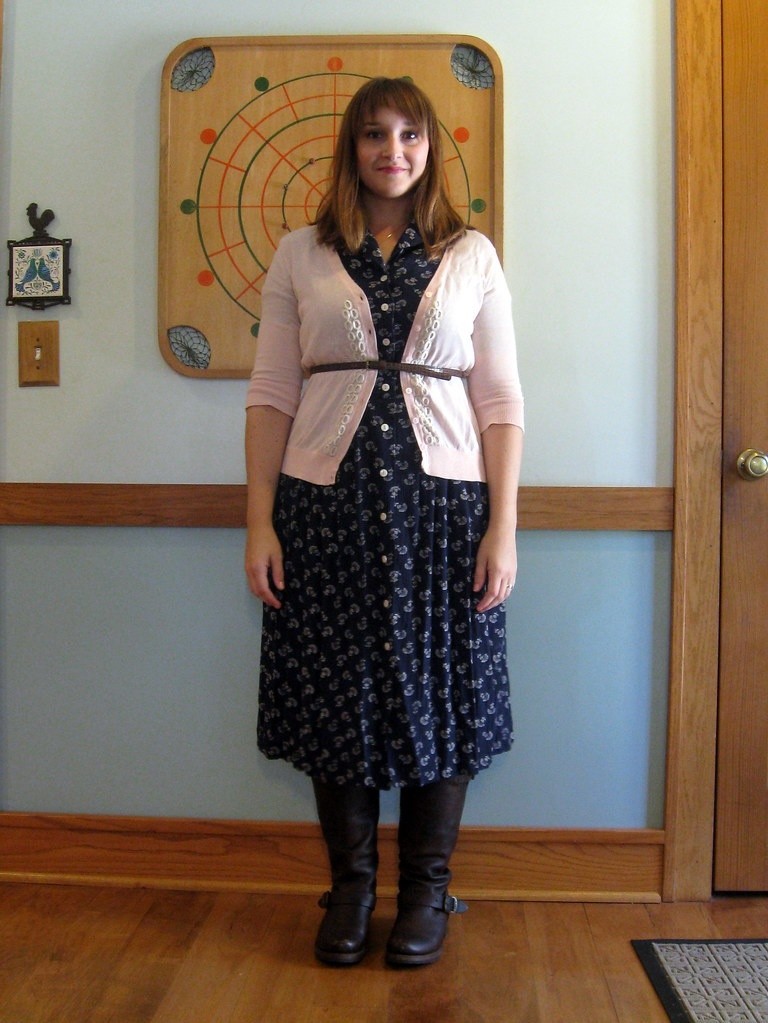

630,938,768,1023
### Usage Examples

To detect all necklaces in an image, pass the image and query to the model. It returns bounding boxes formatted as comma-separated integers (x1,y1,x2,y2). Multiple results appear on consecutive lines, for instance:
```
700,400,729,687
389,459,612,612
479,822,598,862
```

375,222,407,246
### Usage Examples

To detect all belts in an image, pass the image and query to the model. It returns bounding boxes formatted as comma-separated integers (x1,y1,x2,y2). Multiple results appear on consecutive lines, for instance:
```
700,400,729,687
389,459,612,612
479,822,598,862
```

309,362,467,381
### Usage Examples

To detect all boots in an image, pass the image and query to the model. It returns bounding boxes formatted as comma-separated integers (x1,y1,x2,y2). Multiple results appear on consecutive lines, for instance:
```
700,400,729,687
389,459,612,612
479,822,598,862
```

385,778,470,963
310,778,380,963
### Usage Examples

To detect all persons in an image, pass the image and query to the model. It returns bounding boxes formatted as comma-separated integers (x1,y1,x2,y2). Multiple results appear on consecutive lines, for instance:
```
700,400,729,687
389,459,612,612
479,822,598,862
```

242,77,526,967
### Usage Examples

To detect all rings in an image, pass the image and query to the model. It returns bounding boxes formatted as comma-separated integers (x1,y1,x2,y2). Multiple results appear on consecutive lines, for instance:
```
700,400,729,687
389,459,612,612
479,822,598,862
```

507,585,514,589
